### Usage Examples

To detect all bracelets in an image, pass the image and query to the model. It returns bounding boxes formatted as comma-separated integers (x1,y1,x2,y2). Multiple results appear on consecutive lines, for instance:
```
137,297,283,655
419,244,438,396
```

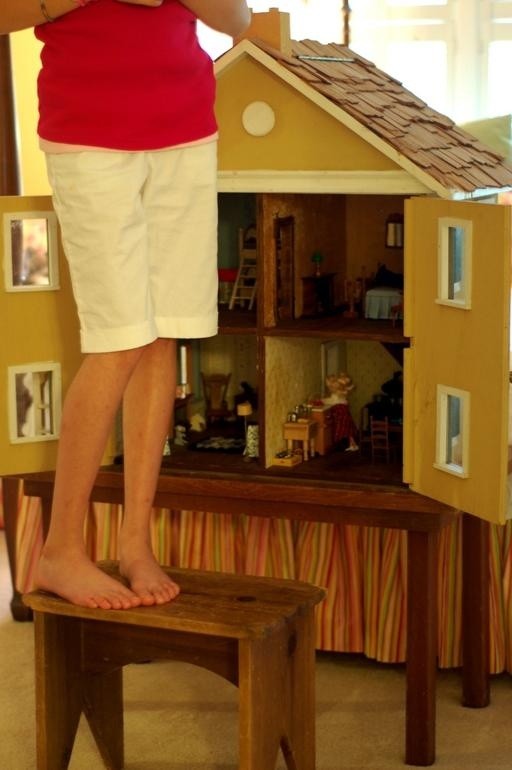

40,0,54,24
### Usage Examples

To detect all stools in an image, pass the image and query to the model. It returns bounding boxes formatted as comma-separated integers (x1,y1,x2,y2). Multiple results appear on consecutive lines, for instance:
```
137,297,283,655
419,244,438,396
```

20,554,328,770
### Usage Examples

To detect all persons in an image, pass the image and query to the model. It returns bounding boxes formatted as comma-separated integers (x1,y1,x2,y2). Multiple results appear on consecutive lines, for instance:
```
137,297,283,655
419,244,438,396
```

0,0,251,612
21,234,46,286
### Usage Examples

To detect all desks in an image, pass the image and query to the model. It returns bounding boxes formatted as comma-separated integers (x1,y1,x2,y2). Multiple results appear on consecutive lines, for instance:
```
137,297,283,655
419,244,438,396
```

1,446,488,769
